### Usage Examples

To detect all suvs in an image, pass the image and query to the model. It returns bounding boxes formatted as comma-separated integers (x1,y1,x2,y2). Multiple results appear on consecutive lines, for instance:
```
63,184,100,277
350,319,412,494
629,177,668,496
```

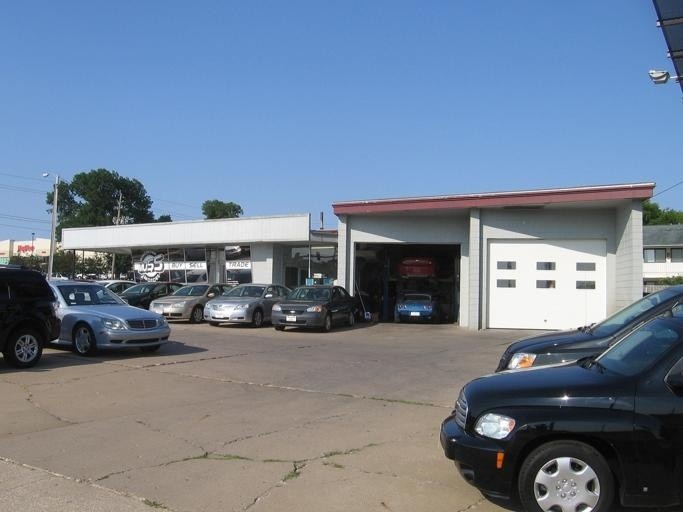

0,265,61,368
494,284,682,373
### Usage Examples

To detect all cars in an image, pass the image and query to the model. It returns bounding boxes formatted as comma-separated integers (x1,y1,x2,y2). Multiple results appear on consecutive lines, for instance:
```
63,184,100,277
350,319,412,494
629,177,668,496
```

93,280,136,296
202,284,291,328
42,271,127,281
42,279,170,357
397,253,442,279
439,315,682,511
113,282,184,311
270,284,357,333
394,288,440,323
148,283,234,323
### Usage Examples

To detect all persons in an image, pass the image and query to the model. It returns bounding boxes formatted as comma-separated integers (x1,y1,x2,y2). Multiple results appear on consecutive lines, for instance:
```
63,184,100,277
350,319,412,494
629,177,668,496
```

140,292,159,310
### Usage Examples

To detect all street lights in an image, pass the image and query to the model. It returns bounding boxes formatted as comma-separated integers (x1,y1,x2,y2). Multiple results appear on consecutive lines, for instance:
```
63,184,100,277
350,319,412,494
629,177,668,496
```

42,171,59,281
